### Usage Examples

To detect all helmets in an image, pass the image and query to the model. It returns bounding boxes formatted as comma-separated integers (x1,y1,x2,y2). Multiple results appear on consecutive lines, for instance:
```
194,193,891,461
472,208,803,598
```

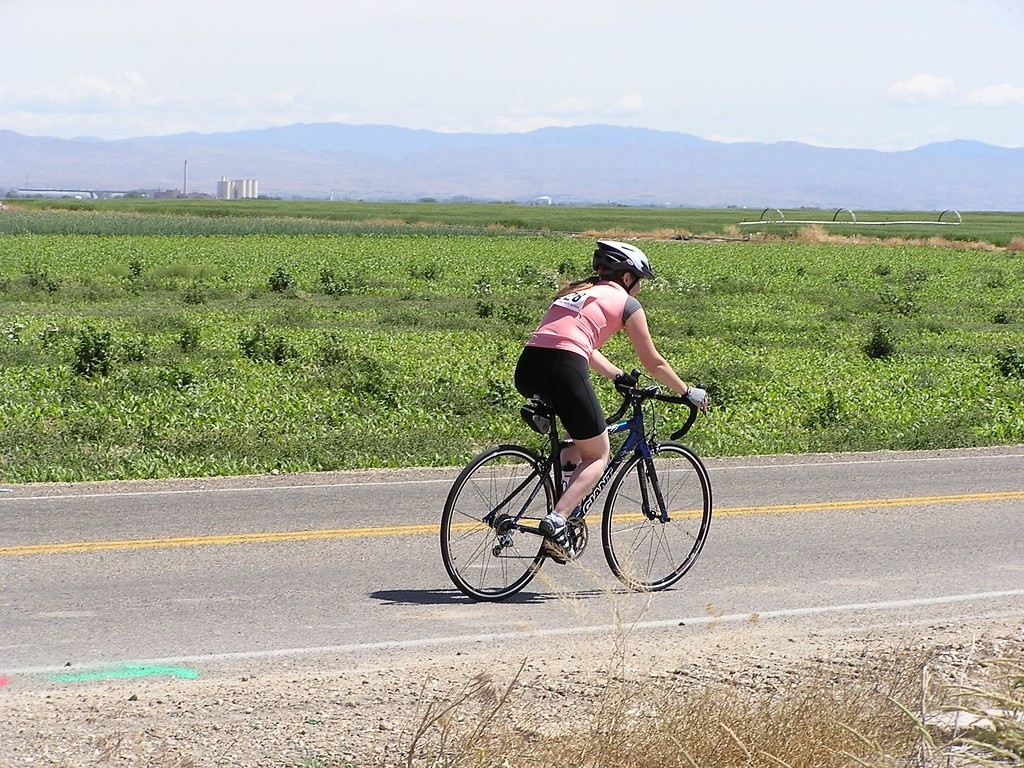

593,240,655,281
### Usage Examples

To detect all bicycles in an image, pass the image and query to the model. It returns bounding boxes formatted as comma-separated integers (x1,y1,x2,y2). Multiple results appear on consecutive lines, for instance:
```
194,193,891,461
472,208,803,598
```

439,368,712,601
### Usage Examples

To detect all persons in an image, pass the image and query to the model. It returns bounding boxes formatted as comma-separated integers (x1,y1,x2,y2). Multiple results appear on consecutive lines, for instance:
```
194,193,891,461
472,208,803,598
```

514,241,709,556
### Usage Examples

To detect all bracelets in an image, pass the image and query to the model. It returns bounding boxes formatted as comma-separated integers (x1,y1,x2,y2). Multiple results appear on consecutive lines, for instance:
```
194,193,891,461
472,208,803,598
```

682,385,691,396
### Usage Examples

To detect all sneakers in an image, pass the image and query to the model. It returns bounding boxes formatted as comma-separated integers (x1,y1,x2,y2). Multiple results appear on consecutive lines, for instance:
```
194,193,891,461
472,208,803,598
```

538,514,577,558
569,503,582,518
